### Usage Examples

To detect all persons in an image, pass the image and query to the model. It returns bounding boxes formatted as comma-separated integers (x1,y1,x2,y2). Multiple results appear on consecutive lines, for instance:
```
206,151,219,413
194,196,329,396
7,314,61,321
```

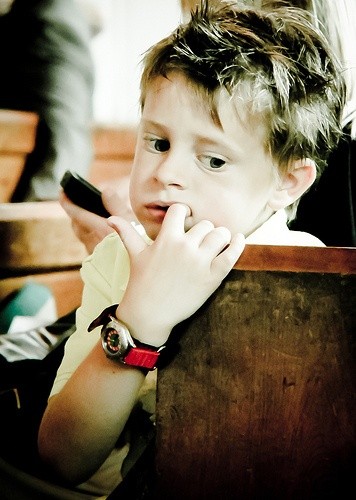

36,0,346,500
56,187,129,256
0,0,95,202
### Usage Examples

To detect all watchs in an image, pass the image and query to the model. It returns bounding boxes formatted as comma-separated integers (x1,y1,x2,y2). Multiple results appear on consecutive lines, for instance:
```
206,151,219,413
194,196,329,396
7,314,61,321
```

86,304,163,372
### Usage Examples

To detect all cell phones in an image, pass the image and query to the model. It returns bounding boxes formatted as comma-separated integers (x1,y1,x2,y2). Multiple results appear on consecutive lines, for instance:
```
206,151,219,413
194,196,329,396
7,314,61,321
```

61,169,111,219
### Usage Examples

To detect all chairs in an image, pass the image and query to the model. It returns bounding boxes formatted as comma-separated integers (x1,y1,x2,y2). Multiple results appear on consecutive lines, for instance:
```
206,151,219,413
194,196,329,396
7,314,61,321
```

148,245,356,500
